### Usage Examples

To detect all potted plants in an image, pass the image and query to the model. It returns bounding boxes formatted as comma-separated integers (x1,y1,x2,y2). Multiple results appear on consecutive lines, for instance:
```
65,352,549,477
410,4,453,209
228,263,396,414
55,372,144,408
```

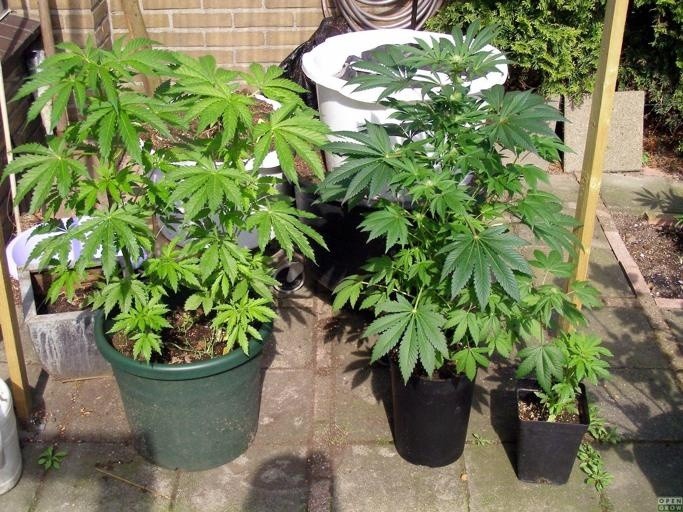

442,247,616,487
313,17,583,468
2,33,332,473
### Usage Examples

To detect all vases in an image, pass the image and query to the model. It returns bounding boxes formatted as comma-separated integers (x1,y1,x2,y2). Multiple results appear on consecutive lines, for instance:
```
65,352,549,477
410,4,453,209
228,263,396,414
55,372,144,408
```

303,29,510,204
6,218,144,283
136,91,292,252
17,256,134,382
294,172,484,311
